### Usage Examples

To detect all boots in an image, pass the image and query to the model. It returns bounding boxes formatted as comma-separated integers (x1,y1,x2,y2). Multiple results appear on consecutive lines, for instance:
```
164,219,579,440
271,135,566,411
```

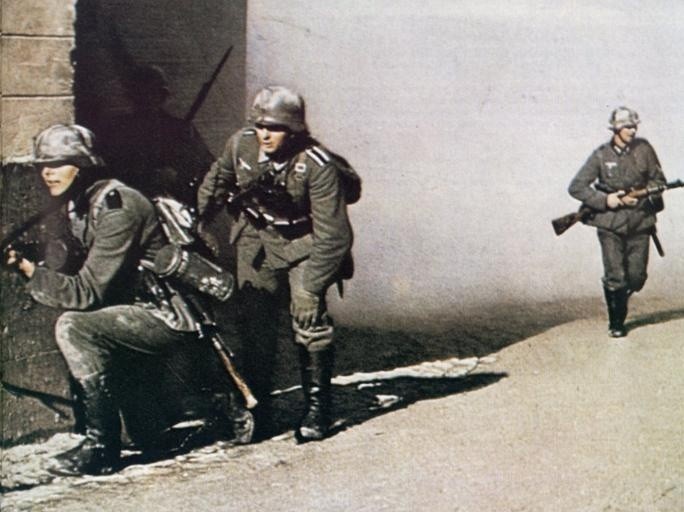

184,370,255,447
43,374,121,475
293,345,338,443
601,284,642,339
222,355,274,439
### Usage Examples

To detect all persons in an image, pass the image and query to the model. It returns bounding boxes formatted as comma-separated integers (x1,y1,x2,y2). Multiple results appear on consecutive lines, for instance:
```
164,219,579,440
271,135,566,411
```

193,85,357,442
565,105,666,339
0,122,255,477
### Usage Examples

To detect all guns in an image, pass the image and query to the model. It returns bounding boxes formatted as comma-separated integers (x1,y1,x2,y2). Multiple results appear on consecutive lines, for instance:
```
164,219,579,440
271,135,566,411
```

552,179,683,236
178,287,259,410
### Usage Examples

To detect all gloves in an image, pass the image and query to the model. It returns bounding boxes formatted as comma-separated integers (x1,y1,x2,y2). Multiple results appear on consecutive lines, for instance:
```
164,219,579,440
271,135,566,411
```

289,290,324,331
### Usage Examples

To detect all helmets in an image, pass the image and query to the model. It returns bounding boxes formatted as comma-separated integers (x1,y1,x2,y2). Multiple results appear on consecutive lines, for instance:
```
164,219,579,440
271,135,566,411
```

246,86,311,139
607,106,644,131
31,125,105,169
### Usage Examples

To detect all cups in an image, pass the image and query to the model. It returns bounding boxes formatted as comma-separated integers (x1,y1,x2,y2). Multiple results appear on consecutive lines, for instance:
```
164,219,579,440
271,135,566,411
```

154,246,234,301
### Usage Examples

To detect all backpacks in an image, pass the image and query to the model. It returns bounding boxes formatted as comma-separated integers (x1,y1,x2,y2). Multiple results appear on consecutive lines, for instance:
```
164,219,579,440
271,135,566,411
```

242,132,361,205
62,181,227,276
